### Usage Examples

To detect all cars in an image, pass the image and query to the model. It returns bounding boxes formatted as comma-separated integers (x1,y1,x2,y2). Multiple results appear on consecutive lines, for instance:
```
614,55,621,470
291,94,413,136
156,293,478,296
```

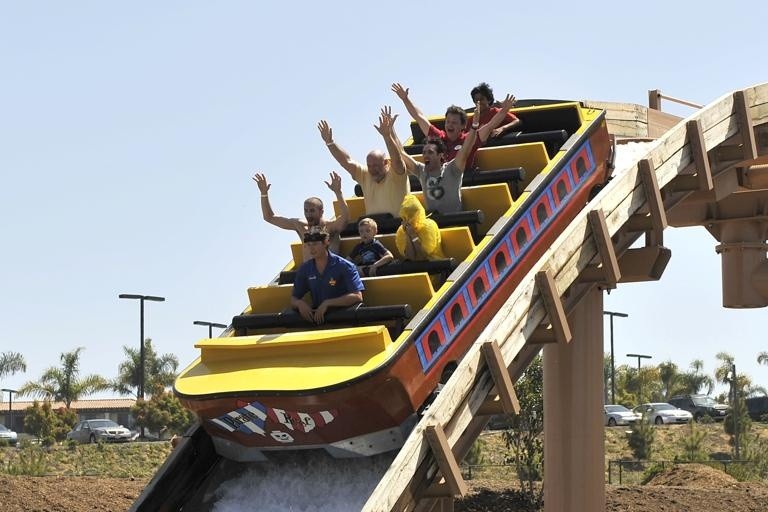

630,402,694,425
0,422,18,449
66,419,133,443
602,404,643,427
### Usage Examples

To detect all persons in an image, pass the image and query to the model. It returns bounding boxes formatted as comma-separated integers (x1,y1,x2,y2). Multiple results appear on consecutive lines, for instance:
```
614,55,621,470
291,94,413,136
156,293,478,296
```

319,120,412,221
252,171,350,264
380,100,481,213
290,224,365,325
345,217,394,278
390,81,517,170
464,82,522,138
394,192,446,292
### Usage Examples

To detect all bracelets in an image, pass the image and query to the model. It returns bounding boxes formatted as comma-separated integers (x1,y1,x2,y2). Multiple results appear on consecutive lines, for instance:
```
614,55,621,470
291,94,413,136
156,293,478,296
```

469,123,480,131
327,140,335,145
412,236,419,243
261,194,268,198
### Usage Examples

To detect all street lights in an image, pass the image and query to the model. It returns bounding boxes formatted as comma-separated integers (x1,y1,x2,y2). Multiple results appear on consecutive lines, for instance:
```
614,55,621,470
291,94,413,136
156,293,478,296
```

604,308,626,404
193,320,229,340
626,354,654,403
1,388,17,410
119,292,166,436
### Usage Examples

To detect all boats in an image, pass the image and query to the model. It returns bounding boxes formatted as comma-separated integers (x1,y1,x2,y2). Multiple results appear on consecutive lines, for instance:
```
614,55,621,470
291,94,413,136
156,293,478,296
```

170,97,611,463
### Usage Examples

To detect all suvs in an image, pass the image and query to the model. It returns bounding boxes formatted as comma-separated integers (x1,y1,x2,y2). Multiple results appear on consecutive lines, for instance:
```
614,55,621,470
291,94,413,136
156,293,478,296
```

668,393,732,423
